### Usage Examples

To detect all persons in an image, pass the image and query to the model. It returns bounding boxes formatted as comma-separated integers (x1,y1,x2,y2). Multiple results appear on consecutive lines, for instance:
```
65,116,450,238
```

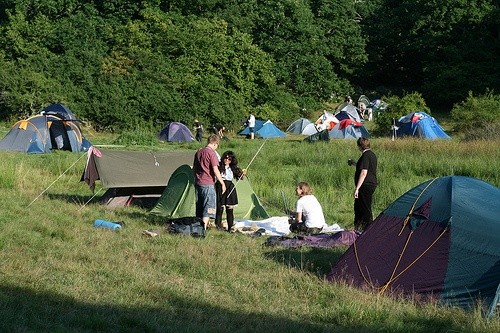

248,111,256,139
194,135,227,231
347,137,379,233
283,181,325,237
211,121,227,139
213,151,247,233
194,118,203,142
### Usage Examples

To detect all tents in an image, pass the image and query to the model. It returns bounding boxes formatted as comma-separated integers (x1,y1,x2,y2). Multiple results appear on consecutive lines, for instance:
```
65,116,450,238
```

156,122,198,143
285,95,390,143
150,164,272,222
321,175,500,320
1,103,93,156
391,112,451,140
238,119,288,138
79,144,223,210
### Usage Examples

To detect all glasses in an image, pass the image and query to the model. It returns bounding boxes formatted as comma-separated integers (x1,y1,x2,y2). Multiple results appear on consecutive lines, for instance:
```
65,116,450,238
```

225,156,232,161
